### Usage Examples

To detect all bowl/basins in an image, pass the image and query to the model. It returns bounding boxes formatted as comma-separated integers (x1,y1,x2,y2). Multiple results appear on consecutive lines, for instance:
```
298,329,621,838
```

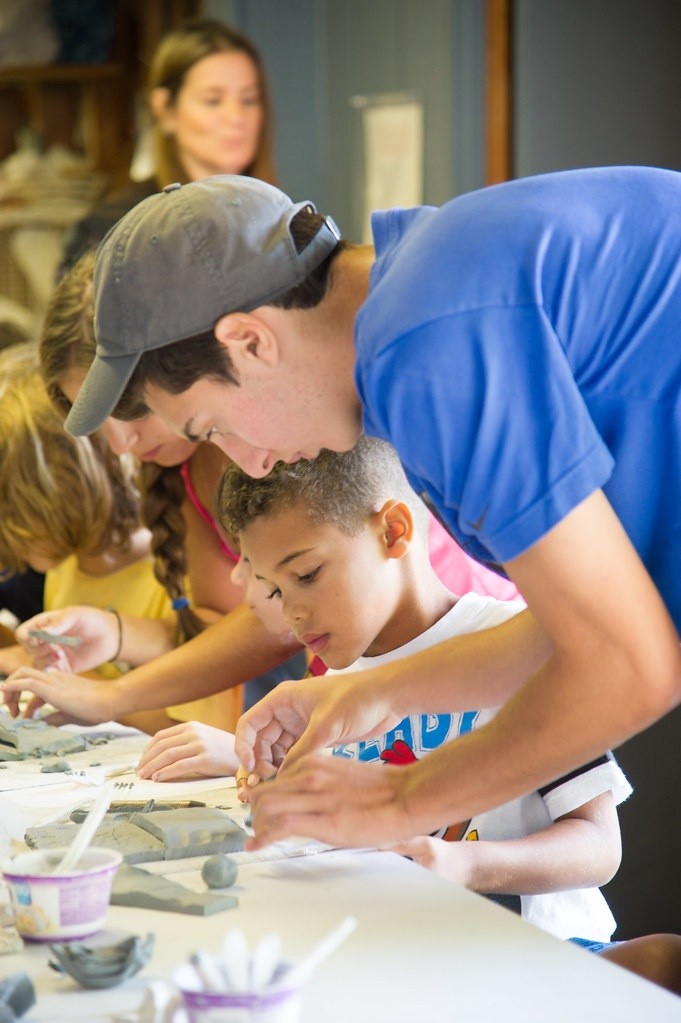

1,848,123,944
173,949,313,1023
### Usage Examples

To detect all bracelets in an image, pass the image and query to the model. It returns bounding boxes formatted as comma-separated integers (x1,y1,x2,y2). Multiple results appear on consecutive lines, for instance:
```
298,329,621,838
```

106,608,121,662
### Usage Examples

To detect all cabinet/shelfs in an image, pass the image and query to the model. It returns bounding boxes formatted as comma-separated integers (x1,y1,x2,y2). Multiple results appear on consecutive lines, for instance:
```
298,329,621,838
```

0,0,187,201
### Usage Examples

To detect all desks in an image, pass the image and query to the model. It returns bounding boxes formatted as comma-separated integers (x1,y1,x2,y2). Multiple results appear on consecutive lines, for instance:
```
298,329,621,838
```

0,701,681,1023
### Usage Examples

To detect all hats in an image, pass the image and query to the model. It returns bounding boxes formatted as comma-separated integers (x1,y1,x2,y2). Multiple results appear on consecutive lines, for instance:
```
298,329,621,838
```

64,175,341,436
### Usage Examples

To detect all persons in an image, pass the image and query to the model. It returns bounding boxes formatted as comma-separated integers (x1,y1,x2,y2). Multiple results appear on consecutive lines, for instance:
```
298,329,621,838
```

137,21,274,187
566,933,681,996
63,166,681,845
0,243,634,942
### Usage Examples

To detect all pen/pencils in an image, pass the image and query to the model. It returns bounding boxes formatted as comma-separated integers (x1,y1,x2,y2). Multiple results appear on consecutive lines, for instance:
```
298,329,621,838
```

110,800,207,811
